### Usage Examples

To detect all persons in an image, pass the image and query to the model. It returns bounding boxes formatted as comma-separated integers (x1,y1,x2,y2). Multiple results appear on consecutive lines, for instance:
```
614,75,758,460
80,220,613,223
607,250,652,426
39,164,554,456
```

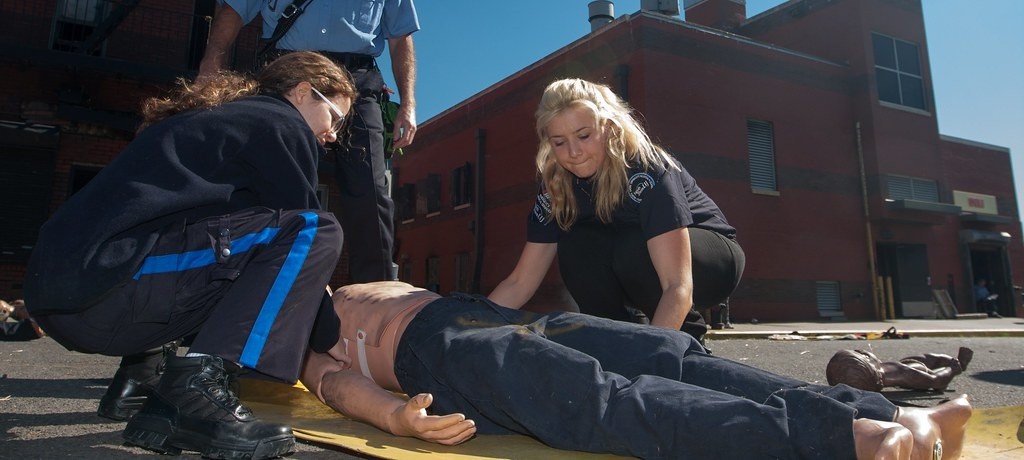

300,281,973,460
24,50,355,460
488,79,746,355
827,346,974,392
197,0,420,283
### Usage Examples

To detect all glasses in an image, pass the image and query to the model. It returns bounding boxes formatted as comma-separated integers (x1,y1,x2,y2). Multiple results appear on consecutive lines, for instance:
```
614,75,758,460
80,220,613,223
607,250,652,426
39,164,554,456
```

289,83,345,133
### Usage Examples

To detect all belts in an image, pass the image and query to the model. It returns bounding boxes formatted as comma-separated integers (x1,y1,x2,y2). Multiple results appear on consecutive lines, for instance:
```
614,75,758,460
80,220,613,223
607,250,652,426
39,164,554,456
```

274,47,373,70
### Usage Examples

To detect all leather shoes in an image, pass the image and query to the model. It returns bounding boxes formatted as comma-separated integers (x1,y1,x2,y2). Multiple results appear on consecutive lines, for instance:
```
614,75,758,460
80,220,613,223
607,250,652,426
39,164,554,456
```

97,338,182,420
119,352,295,459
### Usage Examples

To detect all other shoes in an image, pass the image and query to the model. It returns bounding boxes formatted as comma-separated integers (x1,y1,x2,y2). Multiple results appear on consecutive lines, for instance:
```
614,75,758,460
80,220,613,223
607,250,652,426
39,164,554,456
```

711,322,724,329
724,323,733,329
